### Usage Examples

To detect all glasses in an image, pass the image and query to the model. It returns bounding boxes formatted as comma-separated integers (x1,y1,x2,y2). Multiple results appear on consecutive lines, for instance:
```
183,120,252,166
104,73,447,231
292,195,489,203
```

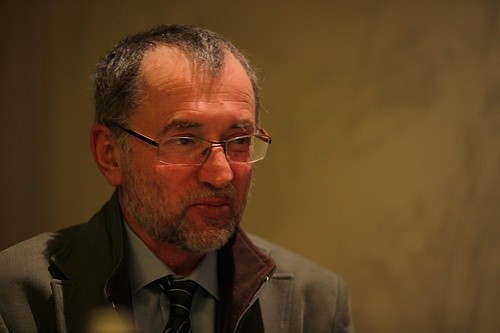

111,126,272,166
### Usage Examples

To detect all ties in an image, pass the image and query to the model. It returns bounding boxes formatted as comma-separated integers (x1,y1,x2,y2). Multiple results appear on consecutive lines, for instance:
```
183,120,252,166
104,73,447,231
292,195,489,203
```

162,280,198,333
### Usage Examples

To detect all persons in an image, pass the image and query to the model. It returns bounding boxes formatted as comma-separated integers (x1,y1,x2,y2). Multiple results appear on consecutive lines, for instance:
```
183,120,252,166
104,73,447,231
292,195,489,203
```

0,22,354,333
